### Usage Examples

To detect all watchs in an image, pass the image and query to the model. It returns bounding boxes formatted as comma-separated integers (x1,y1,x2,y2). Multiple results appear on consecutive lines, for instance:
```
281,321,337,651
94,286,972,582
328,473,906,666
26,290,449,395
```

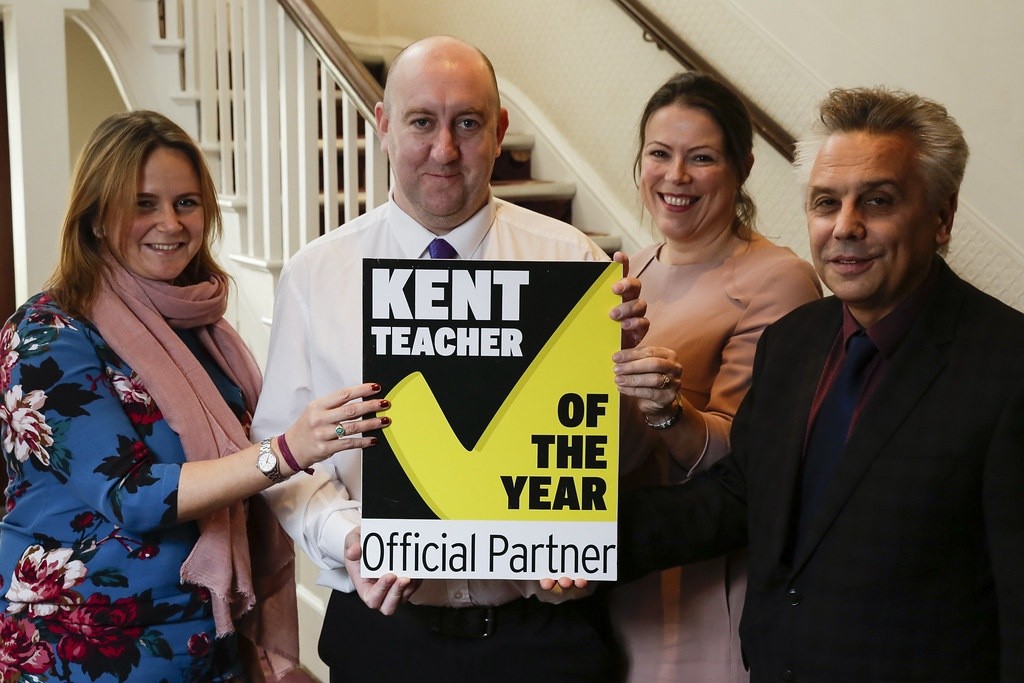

644,390,683,430
255,436,290,483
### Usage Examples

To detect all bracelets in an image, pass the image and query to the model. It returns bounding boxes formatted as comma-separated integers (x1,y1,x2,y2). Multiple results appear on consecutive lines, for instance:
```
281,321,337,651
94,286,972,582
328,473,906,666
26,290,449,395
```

277,434,315,475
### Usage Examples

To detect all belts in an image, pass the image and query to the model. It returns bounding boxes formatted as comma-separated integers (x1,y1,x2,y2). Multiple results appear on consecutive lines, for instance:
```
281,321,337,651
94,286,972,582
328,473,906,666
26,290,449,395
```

407,596,533,637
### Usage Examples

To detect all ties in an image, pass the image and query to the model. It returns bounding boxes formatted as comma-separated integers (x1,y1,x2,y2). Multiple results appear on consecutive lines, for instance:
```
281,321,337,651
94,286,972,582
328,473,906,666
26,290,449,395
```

792,334,876,553
427,239,457,259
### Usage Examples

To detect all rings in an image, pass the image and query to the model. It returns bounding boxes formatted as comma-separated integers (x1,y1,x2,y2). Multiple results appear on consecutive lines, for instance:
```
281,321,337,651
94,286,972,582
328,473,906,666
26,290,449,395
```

335,423,344,438
659,372,670,388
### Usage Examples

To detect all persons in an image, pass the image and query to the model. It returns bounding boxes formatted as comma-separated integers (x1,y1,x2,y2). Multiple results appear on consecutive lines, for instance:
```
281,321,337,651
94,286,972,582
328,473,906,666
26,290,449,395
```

250,35,650,683
602,70,826,683
537,82,1024,680
0,108,392,683
